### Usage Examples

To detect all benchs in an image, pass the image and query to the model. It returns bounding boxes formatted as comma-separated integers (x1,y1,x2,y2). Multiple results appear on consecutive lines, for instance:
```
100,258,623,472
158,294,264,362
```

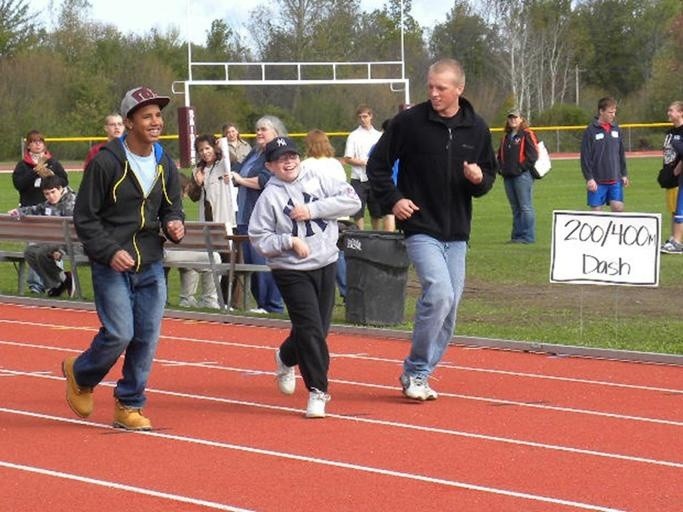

1,212,273,314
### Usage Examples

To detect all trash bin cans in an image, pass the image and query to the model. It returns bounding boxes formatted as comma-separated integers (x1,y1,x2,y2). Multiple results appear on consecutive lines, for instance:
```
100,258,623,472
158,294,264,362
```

337,228,410,328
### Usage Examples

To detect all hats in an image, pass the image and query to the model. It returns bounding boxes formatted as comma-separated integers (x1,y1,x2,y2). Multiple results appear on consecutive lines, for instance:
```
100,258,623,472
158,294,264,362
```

507,109,519,117
121,87,170,121
265,137,301,161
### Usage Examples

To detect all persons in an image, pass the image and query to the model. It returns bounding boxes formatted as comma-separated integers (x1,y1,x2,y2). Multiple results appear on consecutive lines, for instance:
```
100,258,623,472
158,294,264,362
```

496,109,539,246
661,148,683,256
364,58,499,402
7,173,78,300
579,97,630,214
657,100,683,249
245,134,365,420
13,131,70,296
82,103,410,315
63,87,187,433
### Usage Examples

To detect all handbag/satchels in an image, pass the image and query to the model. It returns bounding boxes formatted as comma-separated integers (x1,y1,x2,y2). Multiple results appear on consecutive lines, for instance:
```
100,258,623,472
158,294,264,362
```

528,140,551,179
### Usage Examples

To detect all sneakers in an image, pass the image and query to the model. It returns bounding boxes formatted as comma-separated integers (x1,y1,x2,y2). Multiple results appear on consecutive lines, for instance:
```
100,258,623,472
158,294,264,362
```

504,238,532,244
30,286,40,294
48,282,66,296
277,351,296,395
113,398,152,431
306,387,331,418
426,385,438,400
176,293,244,315
400,373,426,400
60,271,76,298
249,306,270,315
661,240,683,254
61,358,93,418
